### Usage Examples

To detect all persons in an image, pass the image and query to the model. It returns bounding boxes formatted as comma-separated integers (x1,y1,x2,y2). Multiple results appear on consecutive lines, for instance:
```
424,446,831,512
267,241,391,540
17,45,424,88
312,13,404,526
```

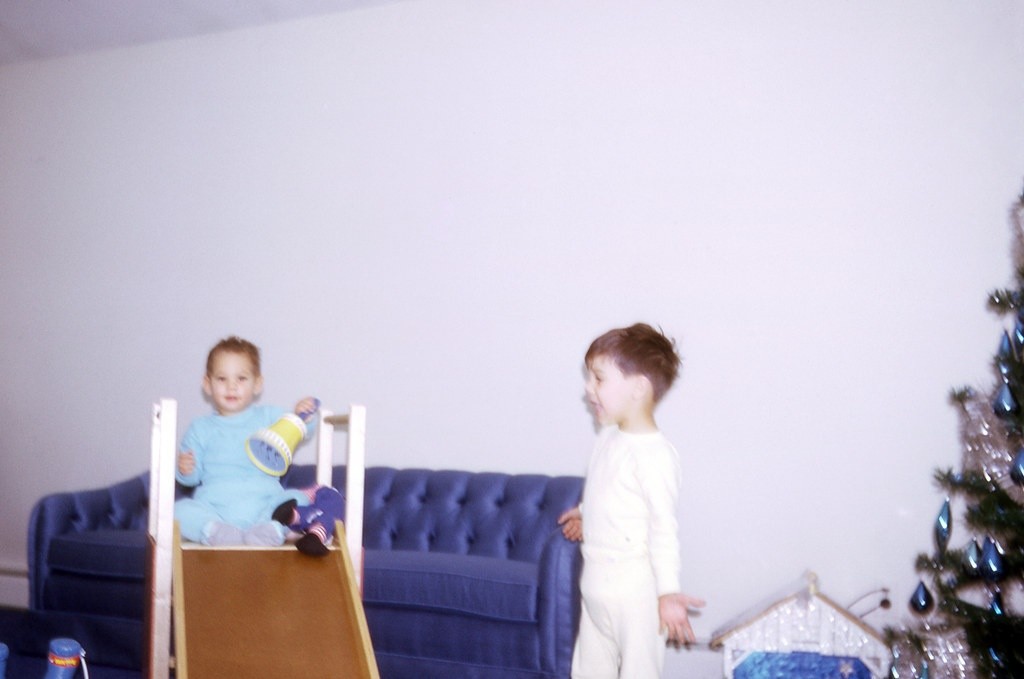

557,322,708,679
172,336,319,546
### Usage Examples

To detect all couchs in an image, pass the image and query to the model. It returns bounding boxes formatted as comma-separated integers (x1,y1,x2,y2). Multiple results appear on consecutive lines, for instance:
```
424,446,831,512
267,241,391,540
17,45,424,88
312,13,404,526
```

26,464,587,679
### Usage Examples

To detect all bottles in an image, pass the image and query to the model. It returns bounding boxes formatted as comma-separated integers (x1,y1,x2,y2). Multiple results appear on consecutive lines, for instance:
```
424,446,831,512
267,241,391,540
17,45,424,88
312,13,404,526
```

45,639,82,679
0,643,8,679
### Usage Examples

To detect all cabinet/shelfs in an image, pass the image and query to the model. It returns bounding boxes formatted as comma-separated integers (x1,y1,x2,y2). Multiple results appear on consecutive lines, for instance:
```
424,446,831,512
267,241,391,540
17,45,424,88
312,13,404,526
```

146,396,380,679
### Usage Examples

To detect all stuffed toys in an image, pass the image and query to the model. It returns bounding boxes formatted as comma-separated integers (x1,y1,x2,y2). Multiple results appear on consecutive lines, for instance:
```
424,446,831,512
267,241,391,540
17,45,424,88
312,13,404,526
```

276,482,347,555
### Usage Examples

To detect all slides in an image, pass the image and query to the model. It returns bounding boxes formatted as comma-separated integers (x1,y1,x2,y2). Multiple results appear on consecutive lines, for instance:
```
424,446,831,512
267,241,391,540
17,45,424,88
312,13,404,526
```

166,514,386,679
